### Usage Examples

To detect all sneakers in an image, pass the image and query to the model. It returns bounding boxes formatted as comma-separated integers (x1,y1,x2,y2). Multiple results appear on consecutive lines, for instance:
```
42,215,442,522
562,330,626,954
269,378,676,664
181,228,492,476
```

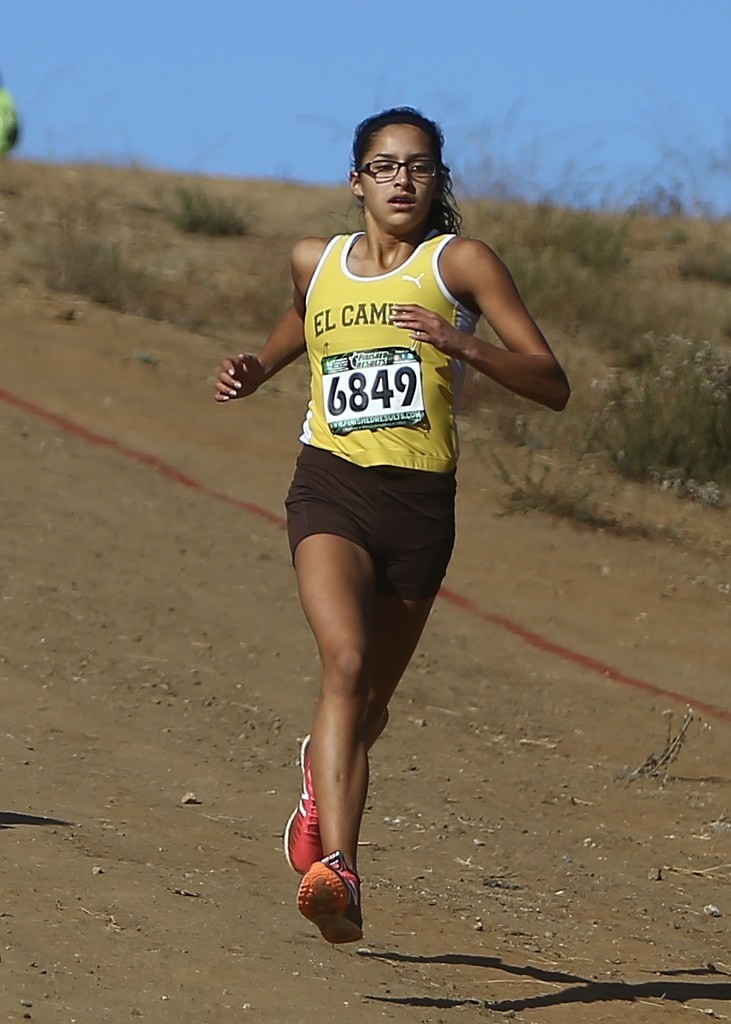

284,734,325,874
296,851,362,944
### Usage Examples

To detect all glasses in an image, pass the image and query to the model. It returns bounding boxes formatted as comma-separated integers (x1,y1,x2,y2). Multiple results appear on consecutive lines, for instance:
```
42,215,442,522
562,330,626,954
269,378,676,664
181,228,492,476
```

358,159,438,178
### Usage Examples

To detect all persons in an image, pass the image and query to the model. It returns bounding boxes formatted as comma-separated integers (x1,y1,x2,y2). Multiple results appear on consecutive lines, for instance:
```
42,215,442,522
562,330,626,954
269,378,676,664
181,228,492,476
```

214,107,569,945
